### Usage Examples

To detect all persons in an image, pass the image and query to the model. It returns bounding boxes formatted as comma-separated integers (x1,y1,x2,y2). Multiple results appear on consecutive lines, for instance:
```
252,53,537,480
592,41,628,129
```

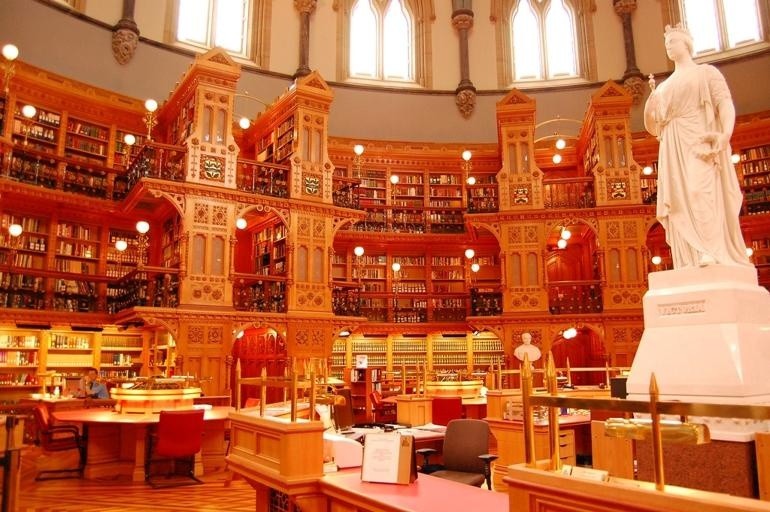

514,332,542,363
643,23,754,270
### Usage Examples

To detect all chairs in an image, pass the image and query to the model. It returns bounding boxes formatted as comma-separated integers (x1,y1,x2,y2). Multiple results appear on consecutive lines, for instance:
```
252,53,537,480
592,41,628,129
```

145,408,205,490
31,404,85,481
416,419,499,490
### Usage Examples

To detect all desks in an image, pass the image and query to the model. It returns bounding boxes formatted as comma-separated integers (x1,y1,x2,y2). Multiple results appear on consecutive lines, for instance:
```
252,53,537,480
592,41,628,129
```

336,422,449,472
49,405,236,484
18,394,232,446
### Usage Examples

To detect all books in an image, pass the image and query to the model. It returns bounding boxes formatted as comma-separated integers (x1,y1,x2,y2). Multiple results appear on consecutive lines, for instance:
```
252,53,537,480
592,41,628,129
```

252,217,289,312
329,333,508,397
1,96,157,201
0,213,182,312
1,329,143,395
639,146,770,216
328,252,502,325
170,97,193,146
149,328,176,378
254,116,294,196
328,165,500,234
582,130,600,171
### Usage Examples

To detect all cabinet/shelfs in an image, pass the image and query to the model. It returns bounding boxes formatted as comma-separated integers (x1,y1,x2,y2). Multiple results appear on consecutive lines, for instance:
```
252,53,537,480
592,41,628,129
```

0,46,770,381
